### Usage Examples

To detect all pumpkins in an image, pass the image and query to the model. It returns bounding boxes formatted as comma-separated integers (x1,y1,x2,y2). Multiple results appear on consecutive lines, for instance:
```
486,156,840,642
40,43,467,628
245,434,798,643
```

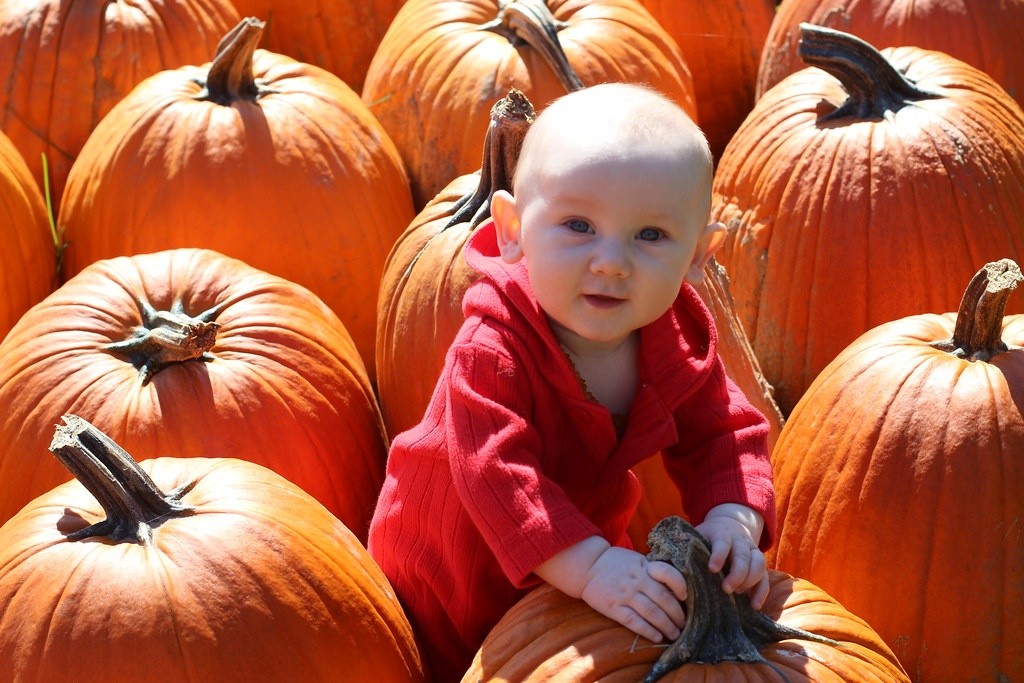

0,0,1024,683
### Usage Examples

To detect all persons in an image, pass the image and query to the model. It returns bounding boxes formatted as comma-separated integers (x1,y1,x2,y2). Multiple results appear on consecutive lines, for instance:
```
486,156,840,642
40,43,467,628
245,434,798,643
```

370,83,779,683
